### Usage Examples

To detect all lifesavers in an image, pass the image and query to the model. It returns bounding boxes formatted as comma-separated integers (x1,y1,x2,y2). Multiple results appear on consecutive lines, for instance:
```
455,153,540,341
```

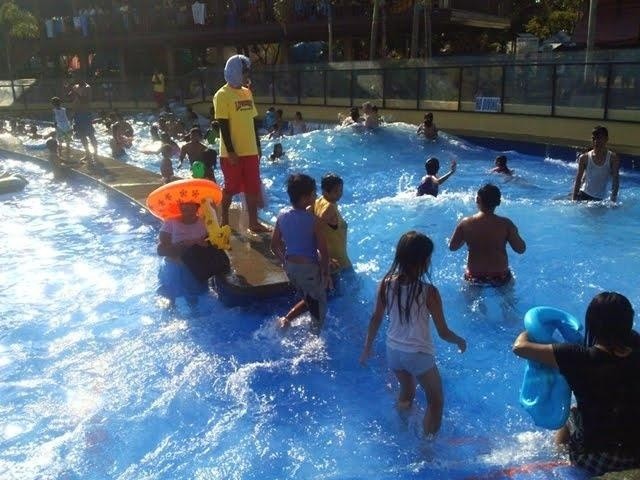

146,178,233,251
0,176,25,195
23,138,47,150
519,306,585,429
